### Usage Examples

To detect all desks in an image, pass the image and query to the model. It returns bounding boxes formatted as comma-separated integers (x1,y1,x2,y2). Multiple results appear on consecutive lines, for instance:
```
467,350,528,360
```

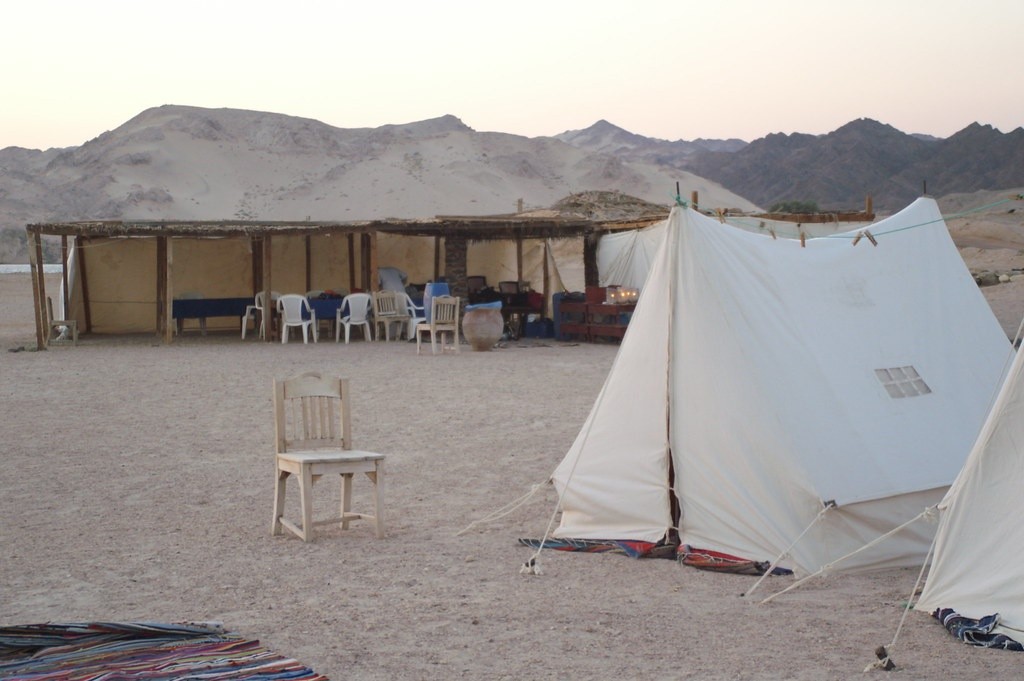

302,298,424,318
172,297,258,337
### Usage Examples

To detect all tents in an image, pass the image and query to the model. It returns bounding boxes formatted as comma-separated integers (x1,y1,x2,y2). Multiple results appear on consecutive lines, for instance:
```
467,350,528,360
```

914,325,1024,652
550,196,1021,586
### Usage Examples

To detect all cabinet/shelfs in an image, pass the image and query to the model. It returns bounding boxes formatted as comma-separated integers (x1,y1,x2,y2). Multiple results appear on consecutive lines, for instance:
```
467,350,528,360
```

559,302,588,341
587,303,635,344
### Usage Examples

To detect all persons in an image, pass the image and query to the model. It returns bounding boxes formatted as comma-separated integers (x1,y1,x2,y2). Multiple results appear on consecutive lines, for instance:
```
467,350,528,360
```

377,266,413,340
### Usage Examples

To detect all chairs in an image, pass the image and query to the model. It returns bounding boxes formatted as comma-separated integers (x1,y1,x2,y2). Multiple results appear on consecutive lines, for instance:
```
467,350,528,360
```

335,293,370,344
255,290,280,339
371,289,412,342
45,296,77,348
416,295,462,356
399,292,427,341
271,370,386,543
467,276,545,335
277,294,318,345
